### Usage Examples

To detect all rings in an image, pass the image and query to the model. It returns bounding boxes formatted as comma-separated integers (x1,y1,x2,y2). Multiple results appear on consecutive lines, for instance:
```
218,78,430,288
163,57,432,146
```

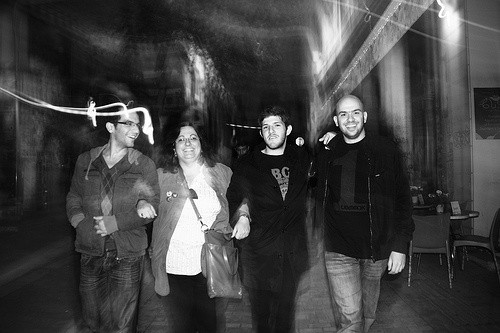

140,213,144,217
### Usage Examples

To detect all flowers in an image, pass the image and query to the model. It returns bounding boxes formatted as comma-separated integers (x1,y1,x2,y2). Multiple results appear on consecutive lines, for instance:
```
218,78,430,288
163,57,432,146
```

409,184,448,204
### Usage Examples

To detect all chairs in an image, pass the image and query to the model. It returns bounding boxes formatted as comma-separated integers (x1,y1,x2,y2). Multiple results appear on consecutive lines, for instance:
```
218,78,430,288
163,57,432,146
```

407,213,454,289
451,208,500,282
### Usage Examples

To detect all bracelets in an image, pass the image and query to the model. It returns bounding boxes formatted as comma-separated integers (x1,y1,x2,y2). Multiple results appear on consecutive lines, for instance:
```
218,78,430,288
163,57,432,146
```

239,213,250,222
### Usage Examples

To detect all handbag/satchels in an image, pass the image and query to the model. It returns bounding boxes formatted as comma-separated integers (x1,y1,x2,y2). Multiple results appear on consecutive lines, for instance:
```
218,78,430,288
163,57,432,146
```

204,229,243,299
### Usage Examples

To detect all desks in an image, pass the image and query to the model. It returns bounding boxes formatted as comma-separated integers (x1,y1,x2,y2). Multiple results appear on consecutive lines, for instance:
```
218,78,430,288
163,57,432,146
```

449,211,479,272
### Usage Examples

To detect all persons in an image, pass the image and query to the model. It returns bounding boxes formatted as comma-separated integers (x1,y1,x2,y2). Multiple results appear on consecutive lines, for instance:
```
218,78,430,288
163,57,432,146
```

136,121,253,333
231,140,250,172
307,94,415,333
66,104,160,333
226,104,337,333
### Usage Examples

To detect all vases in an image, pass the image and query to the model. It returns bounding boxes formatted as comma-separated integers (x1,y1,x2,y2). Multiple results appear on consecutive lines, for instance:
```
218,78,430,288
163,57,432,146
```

412,196,418,206
435,204,445,215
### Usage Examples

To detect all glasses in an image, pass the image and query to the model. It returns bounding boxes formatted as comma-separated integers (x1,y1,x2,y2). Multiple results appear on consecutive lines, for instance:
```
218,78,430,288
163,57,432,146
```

175,135,200,144
110,121,141,130
